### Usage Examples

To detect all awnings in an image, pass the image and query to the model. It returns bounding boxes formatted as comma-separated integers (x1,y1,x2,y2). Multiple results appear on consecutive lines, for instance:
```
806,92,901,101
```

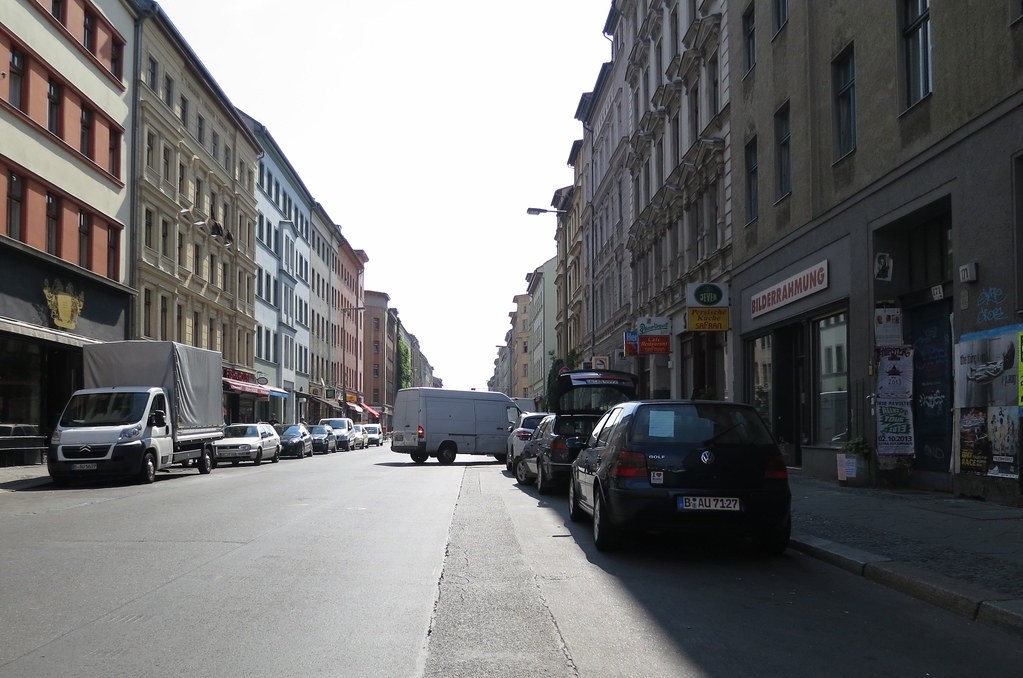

359,403,379,417
313,397,343,411
222,377,289,399
346,402,363,412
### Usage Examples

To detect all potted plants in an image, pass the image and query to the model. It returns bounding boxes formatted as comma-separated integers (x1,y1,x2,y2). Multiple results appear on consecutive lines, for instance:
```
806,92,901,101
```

835,436,872,487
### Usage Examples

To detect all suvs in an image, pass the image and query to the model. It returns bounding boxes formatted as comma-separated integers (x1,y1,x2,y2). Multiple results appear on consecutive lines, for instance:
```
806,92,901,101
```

506,411,555,475
274,423,313,458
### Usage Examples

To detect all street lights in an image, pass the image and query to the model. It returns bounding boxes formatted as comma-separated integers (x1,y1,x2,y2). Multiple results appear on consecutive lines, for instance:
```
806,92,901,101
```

527,208,569,363
343,307,367,417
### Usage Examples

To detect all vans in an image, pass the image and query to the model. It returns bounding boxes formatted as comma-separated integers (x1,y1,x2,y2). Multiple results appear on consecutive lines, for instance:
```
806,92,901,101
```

391,387,530,467
318,418,355,452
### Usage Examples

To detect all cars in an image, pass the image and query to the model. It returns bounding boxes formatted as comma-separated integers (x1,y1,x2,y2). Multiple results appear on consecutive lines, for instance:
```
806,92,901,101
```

212,421,281,466
307,425,338,454
568,400,793,570
515,414,603,495
364,425,384,446
353,425,369,449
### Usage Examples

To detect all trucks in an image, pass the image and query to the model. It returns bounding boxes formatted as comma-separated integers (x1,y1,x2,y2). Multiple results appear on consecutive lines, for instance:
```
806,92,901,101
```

553,369,641,414
48,340,225,482
